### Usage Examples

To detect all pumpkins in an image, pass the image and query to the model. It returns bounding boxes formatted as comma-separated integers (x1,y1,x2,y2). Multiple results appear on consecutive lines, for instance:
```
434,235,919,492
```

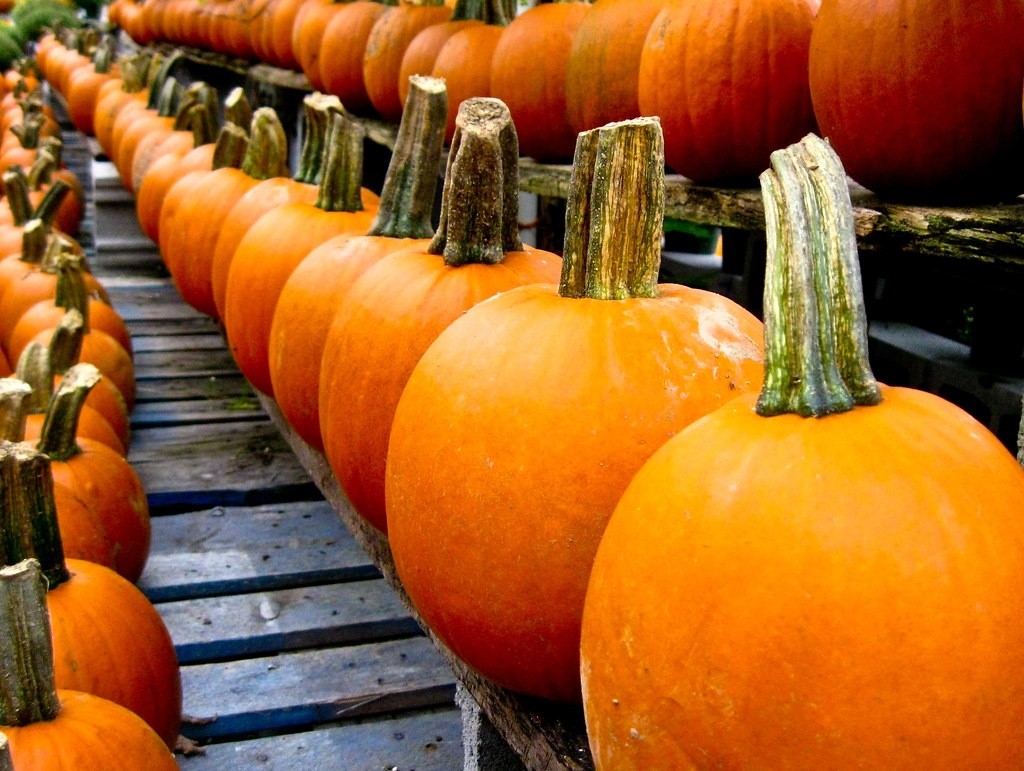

0,0,1024,770
